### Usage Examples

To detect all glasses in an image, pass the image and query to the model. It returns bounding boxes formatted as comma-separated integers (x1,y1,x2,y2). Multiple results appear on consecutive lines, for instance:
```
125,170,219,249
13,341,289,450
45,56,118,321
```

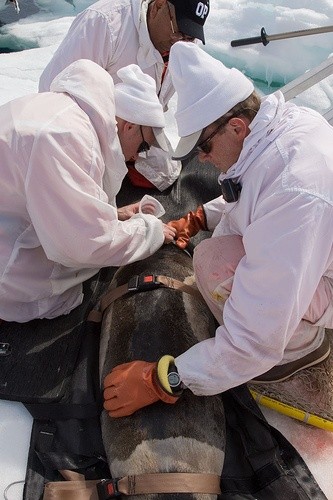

196,106,258,154
165,1,196,43
137,125,150,153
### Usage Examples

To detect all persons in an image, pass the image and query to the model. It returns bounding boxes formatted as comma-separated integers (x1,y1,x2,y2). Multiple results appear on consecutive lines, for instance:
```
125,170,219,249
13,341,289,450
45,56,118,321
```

0,59,177,324
40,0,210,193
103,41,333,418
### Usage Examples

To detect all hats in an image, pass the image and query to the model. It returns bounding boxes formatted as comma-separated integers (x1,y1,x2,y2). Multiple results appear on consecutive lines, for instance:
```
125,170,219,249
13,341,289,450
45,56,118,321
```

168,0,210,46
169,41,254,161
112,64,170,152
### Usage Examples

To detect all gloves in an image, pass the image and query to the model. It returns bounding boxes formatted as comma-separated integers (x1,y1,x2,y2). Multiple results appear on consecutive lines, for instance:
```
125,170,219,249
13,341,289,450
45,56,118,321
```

103,353,183,418
161,204,209,251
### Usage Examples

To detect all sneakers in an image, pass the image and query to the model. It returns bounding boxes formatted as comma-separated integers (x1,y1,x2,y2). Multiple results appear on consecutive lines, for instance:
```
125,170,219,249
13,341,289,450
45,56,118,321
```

244,331,331,384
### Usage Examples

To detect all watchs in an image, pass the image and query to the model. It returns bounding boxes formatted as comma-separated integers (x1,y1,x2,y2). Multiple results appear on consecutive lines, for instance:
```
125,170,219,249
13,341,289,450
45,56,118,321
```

167,364,182,394
